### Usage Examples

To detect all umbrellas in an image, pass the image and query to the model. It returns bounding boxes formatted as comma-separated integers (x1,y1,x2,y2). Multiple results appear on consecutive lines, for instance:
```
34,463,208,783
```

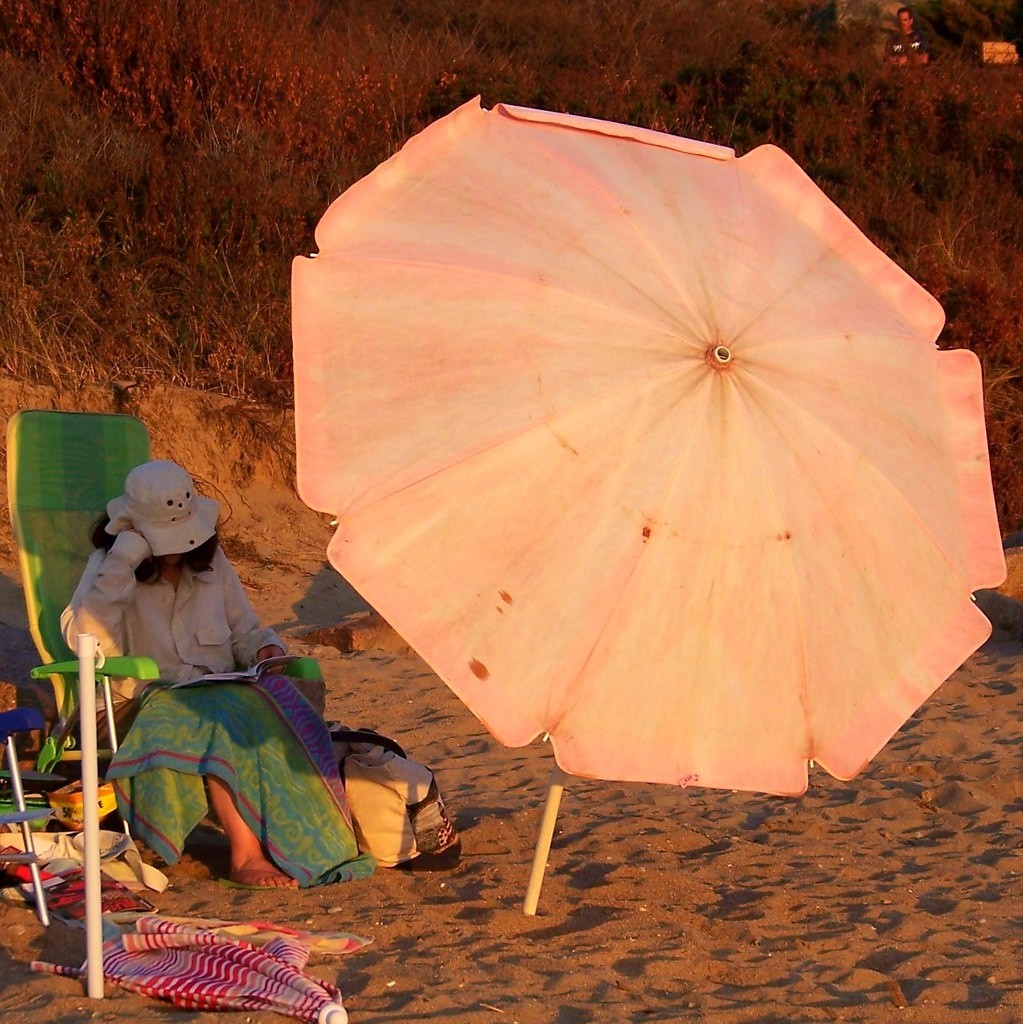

288,95,1011,915
28,916,349,1024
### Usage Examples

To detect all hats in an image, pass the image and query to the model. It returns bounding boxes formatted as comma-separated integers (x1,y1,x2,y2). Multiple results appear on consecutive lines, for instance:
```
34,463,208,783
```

105,460,222,556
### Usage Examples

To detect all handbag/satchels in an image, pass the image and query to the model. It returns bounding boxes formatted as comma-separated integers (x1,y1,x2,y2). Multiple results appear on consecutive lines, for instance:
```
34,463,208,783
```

342,742,458,866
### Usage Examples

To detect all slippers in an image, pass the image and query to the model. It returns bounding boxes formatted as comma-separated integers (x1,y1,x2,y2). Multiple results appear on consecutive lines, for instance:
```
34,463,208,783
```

218,869,299,890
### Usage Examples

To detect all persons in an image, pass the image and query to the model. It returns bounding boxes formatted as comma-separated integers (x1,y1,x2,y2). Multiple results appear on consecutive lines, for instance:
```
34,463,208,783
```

58,460,300,888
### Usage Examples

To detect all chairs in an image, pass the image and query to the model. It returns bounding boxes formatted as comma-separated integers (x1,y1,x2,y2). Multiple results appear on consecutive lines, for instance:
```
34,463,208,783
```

0,707,57,925
7,410,326,836
